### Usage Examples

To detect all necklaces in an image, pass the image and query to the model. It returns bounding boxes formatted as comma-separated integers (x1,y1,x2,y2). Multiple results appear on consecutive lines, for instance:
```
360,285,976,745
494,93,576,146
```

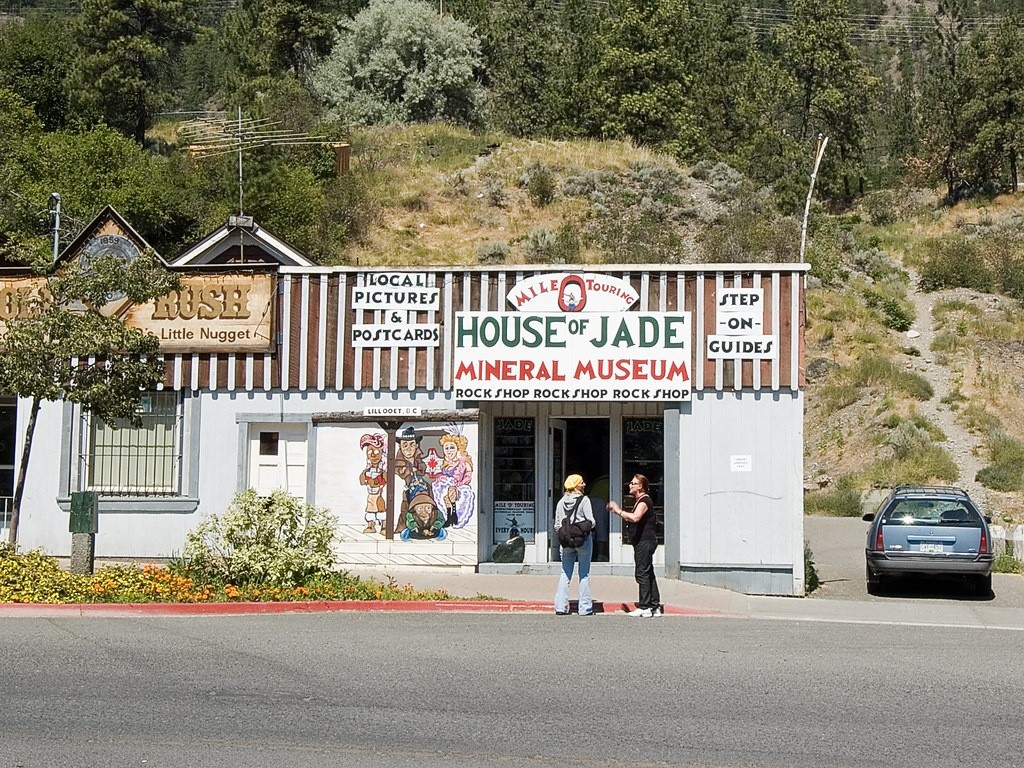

636,493,646,499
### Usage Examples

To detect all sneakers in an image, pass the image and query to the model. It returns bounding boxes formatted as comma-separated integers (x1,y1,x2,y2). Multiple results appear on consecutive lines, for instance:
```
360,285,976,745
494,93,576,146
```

628,607,662,617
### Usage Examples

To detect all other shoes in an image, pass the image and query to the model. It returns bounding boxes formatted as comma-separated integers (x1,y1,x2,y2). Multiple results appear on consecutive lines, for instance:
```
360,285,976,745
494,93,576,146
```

556,609,571,615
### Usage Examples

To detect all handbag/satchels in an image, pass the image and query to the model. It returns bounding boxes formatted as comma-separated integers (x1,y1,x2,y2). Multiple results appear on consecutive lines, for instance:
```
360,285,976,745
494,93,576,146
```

558,517,592,547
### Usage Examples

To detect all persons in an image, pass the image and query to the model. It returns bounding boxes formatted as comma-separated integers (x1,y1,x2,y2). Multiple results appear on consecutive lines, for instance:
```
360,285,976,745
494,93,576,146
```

554,474,596,615
606,474,662,618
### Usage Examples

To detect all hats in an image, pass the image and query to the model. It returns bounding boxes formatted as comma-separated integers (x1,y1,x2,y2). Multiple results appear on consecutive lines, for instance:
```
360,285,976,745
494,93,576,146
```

564,474,583,490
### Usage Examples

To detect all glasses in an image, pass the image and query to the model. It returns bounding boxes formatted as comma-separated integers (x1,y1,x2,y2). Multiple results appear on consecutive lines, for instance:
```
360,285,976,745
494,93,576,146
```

630,481,639,485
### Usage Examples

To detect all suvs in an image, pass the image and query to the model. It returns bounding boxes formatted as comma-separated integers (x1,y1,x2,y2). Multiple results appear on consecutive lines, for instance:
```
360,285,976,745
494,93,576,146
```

861,483,996,595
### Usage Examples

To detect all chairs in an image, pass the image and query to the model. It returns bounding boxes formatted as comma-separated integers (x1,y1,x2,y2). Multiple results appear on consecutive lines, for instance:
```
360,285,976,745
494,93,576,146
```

892,512,913,517
941,510,969,520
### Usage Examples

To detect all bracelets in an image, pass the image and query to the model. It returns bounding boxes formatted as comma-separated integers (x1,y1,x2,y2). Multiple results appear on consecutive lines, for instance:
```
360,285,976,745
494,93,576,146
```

619,511,623,516
